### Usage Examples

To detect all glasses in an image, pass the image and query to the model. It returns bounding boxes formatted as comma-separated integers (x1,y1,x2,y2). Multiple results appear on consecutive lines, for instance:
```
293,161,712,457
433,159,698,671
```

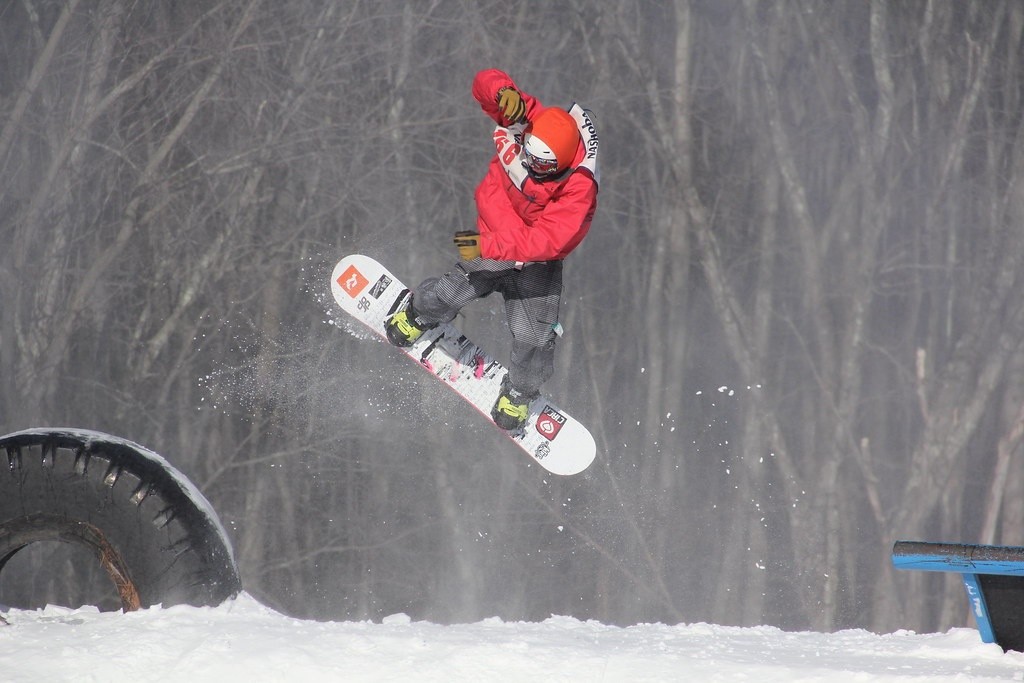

522,148,557,172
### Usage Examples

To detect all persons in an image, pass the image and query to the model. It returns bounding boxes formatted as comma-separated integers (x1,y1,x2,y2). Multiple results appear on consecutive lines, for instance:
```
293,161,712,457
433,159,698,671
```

384,68,599,430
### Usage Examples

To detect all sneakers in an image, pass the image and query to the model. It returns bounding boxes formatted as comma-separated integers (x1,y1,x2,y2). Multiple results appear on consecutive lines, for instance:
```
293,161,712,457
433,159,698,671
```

386,297,438,347
491,367,533,430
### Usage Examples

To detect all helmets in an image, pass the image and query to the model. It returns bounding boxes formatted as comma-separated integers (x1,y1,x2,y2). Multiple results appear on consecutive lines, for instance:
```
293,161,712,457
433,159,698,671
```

524,132,556,159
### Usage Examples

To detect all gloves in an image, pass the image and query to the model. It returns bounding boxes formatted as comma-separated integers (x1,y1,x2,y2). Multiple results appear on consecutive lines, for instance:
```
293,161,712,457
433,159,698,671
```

454,230,481,259
499,87,525,121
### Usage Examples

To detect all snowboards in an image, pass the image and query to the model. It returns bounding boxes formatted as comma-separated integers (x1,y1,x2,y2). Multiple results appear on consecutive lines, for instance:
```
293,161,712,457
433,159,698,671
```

328,254,597,477
